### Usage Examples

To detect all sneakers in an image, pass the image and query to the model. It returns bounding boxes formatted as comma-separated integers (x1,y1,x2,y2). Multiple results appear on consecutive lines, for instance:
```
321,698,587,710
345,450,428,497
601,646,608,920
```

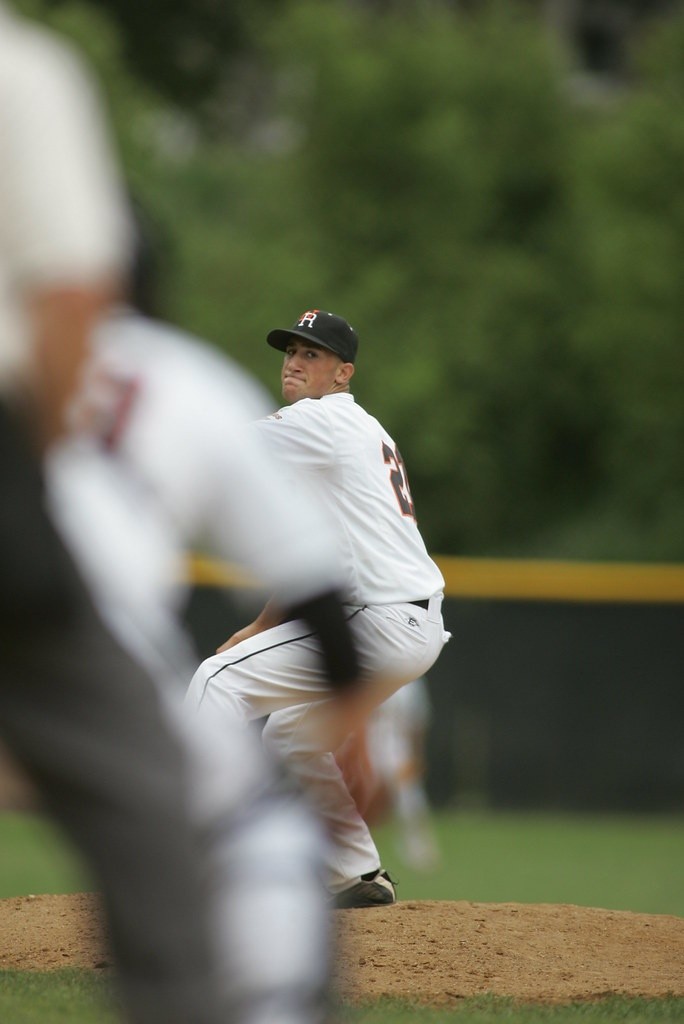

328,870,398,911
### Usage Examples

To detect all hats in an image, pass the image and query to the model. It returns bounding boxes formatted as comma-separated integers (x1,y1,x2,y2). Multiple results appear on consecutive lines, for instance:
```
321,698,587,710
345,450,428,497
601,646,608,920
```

267,310,357,364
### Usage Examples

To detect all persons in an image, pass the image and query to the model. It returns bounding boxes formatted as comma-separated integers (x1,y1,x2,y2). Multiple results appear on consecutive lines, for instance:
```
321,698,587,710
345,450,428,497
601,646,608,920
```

42,204,363,1024
0,13,224,1021
185,308,453,908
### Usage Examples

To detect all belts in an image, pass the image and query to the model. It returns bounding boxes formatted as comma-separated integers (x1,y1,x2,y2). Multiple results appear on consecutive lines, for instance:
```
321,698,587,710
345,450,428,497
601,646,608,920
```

411,598,431,610
